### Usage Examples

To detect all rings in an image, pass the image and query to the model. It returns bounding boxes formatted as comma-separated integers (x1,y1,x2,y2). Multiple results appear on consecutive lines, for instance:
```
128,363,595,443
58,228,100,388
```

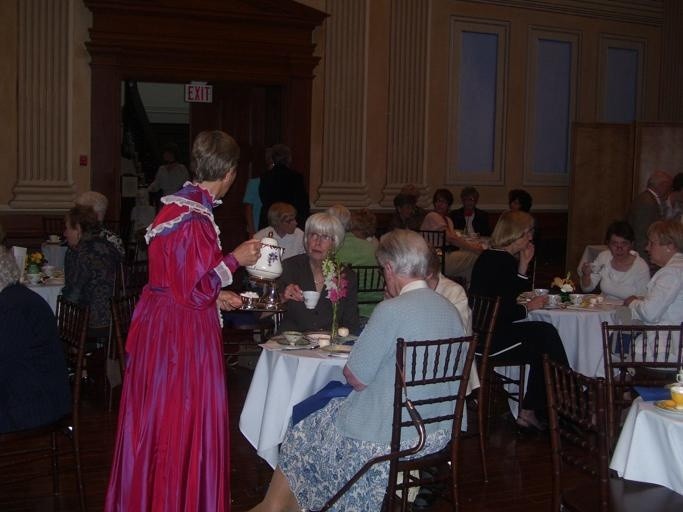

288,293,295,298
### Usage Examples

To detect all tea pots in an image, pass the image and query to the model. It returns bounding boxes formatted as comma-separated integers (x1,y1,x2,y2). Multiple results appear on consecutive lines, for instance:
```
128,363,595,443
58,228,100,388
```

245,231,283,281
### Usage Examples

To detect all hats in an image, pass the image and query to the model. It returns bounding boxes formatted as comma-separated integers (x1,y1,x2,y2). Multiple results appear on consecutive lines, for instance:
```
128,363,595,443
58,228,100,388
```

74,191,108,213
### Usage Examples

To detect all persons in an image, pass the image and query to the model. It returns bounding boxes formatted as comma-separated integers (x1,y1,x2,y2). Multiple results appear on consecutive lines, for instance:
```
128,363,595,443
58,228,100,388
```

101,129,272,512
327,206,385,316
271,210,360,337
380,172,682,441
1,189,122,463
253,202,305,281
244,226,473,510
350,209,382,247
145,152,187,198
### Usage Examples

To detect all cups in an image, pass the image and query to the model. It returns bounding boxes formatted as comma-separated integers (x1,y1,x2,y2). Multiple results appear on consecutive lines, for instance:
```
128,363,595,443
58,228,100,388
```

339,328,348,335
41,265,55,275
534,288,548,296
50,235,59,240
569,294,583,305
548,295,558,306
27,273,40,284
282,332,302,345
671,386,683,409
676,370,683,383
590,263,605,273
303,291,320,310
240,293,260,310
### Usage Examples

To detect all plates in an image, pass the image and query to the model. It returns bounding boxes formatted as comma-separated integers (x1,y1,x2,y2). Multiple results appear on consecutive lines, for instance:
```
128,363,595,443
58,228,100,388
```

47,240,61,242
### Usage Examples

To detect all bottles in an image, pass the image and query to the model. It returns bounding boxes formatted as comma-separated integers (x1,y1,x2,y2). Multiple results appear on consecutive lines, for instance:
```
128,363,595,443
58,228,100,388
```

29,265,38,273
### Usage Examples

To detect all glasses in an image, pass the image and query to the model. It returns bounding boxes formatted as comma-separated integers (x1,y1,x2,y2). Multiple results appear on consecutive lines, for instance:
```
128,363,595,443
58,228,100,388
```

310,232,333,243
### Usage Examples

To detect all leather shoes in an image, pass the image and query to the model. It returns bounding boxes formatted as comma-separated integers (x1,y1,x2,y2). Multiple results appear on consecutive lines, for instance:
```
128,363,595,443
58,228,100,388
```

65,367,89,386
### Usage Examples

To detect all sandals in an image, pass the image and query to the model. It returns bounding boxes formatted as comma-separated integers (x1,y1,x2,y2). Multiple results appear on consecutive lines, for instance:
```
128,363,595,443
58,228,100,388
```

518,415,548,434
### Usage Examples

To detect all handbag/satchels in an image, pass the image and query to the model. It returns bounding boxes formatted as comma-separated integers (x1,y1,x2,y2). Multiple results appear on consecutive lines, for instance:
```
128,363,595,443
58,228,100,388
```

106,356,121,387
